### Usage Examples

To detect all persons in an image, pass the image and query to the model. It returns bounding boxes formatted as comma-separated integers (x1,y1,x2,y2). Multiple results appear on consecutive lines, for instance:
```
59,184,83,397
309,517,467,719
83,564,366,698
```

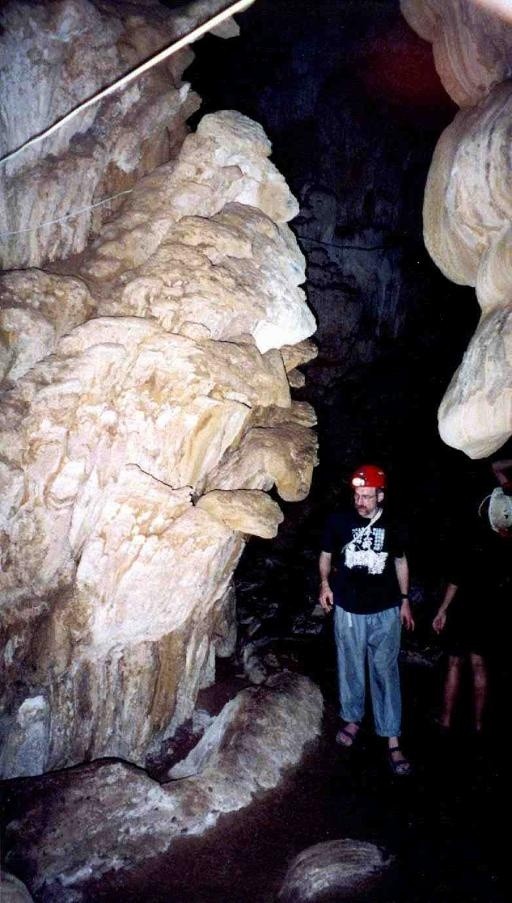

430,551,505,732
317,466,417,776
478,444,512,562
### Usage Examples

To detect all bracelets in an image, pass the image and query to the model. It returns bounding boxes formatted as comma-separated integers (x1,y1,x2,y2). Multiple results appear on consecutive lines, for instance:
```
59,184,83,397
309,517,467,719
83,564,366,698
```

400,594,409,599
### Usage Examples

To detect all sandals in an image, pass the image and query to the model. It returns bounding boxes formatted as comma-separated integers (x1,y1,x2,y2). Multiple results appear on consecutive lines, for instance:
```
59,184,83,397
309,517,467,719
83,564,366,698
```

334,718,362,748
385,743,411,777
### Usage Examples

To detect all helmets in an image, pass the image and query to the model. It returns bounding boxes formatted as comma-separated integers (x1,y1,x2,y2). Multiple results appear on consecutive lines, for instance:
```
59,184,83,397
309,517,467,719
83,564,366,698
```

351,463,386,489
487,487,512,533
